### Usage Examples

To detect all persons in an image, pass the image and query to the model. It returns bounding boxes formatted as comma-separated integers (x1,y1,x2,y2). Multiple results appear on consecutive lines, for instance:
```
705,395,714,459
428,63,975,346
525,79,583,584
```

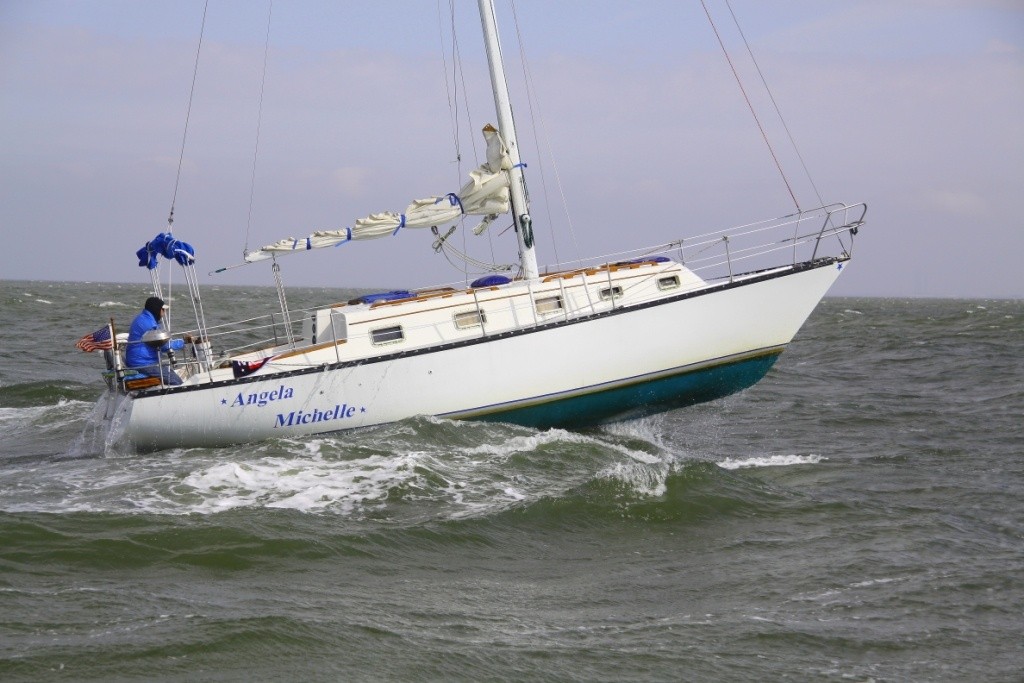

125,297,193,384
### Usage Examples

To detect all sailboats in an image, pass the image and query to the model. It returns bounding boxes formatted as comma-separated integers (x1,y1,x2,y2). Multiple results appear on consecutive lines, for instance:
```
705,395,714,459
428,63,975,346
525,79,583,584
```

75,0,868,453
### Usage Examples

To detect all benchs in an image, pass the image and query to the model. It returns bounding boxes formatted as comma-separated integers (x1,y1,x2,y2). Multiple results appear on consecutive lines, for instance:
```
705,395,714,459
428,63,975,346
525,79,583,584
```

105,369,160,388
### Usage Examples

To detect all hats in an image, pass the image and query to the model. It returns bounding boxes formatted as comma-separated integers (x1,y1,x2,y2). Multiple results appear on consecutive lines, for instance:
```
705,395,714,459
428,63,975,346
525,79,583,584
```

162,304,170,309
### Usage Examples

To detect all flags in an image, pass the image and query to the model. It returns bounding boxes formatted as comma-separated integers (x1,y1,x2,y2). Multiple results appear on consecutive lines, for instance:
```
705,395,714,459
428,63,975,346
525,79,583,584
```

75,325,113,352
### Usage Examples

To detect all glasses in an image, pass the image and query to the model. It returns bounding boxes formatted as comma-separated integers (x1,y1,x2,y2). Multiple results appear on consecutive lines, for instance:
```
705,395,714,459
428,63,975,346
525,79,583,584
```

162,308,165,311
